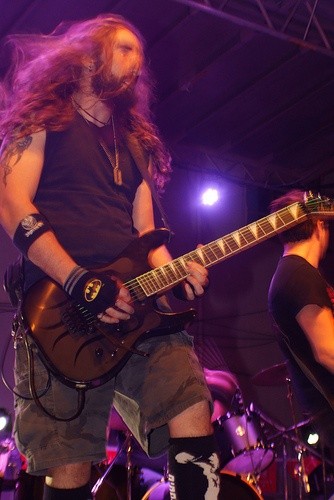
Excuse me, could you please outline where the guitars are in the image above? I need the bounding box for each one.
[20,191,334,391]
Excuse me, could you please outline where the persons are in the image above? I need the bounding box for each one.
[0,15,262,500]
[266,188,334,500]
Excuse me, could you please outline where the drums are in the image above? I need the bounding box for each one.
[212,410,275,475]
[141,469,264,500]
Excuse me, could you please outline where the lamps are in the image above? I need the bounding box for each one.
[0,408,8,430]
[301,425,319,445]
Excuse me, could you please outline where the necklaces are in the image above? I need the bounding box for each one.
[70,91,123,186]
